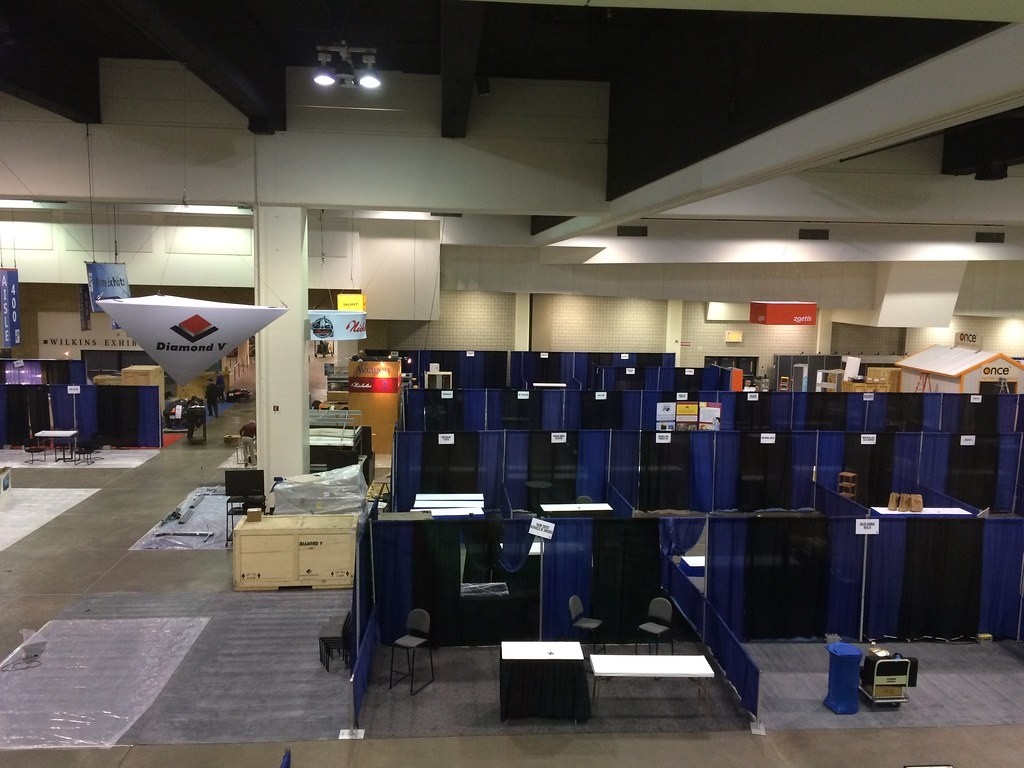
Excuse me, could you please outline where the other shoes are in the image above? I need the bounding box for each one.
[208,414,212,416]
[216,414,218,417]
[245,463,248,467]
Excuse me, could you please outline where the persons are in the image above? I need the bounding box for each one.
[205,371,226,418]
[239,421,257,467]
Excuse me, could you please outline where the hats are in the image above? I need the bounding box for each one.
[208,378,213,382]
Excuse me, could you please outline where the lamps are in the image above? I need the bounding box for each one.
[312,37,384,91]
[473,73,491,97]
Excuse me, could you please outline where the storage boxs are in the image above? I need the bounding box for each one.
[223,434,242,448]
[0,466,11,496]
[862,680,904,699]
[247,508,262,522]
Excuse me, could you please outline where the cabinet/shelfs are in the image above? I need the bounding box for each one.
[225,495,265,548]
[837,470,858,503]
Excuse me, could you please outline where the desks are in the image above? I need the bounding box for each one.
[870,507,972,518]
[35,430,78,463]
[524,480,553,517]
[680,556,706,594]
[588,654,715,722]
[532,382,566,390]
[538,502,613,519]
[499,639,592,726]
[410,493,485,521]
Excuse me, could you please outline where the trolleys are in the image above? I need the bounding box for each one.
[859,658,911,708]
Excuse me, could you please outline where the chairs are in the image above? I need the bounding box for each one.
[319,612,354,672]
[46,428,81,452]
[569,594,607,675]
[576,496,594,504]
[23,439,46,465]
[388,608,436,696]
[74,441,94,465]
[635,596,675,680]
